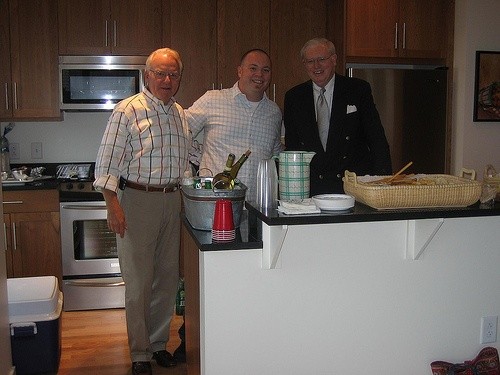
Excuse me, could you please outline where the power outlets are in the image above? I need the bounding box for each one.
[480,315,497,345]
[9,144,20,160]
[31,142,42,159]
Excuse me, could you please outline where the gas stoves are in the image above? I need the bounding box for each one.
[58,178,105,201]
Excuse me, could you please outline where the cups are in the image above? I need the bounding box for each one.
[211,200,236,241]
[256,158,278,208]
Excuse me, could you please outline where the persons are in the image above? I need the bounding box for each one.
[283,37,392,198]
[93,48,194,375]
[183,49,285,210]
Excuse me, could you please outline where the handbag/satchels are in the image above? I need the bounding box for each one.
[430,347,500,375]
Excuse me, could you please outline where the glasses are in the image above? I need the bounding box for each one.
[303,56,331,66]
[149,69,179,81]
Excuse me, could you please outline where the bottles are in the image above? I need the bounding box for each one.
[211,153,235,190]
[231,154,248,180]
[205,179,211,190]
[194,178,202,189]
[176,282,185,315]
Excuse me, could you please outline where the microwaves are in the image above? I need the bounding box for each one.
[58,55,149,112]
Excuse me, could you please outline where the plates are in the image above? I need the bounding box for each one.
[312,194,355,211]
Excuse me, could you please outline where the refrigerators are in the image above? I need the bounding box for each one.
[346,65,449,175]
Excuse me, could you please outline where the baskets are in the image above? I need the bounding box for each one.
[483,165,500,202]
[341,169,482,210]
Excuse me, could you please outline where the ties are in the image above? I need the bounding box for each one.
[316,88,331,152]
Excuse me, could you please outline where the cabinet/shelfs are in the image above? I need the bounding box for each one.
[0,0,456,175]
[2,190,62,291]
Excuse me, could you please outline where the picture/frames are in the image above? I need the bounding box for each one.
[473,50,500,122]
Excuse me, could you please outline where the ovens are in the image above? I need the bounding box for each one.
[60,201,126,312]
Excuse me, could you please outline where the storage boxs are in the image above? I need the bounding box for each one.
[7,276,64,375]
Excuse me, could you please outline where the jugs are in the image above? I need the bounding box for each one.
[272,151,317,201]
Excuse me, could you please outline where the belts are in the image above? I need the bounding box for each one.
[125,180,180,193]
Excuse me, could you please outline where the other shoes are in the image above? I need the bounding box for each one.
[175,341,186,362]
[132,362,152,375]
[152,349,177,366]
